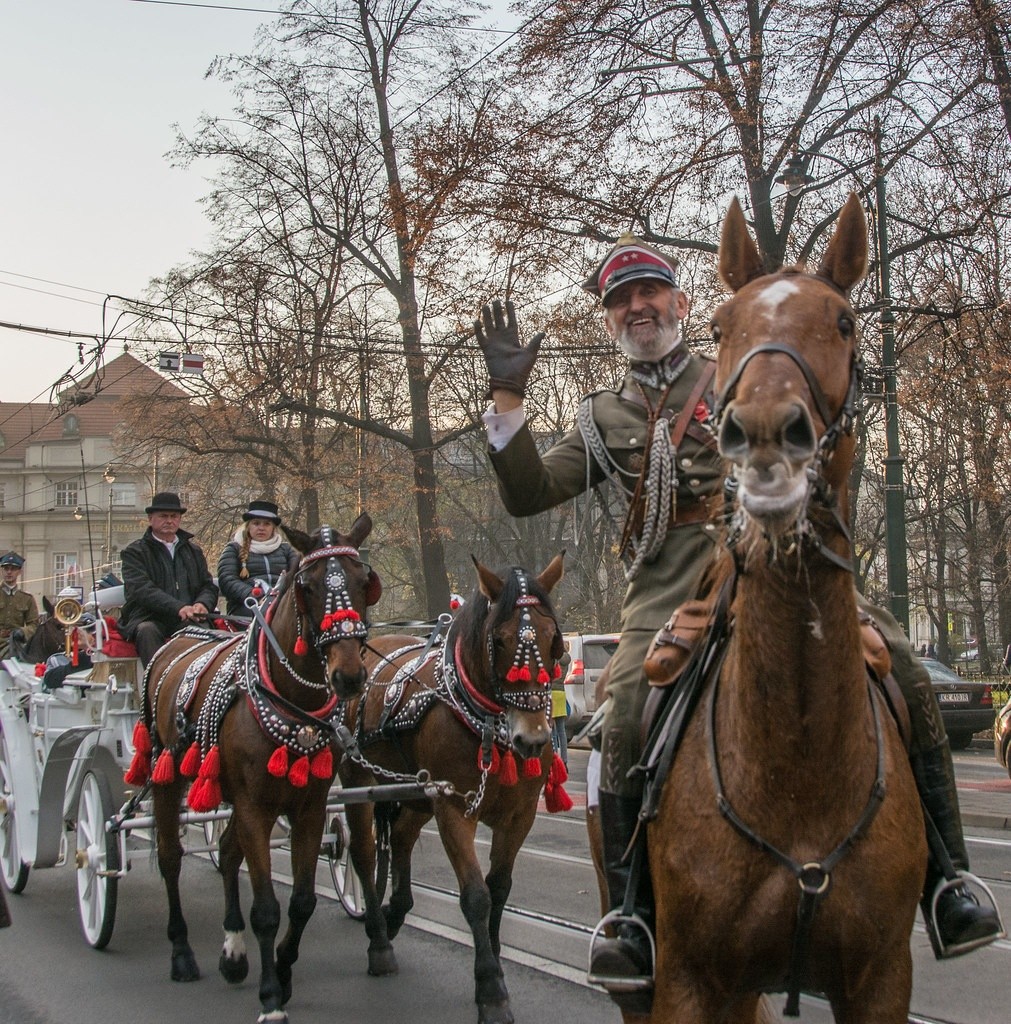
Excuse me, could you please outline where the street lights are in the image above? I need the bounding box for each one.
[773,114,910,642]
[73,487,116,572]
[104,443,160,498]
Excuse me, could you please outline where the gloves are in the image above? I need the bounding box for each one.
[473,299,546,399]
[10,628,25,643]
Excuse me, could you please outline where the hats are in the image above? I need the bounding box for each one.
[0,550,26,568]
[145,491,188,514]
[242,500,283,526]
[94,572,123,588]
[579,231,682,308]
[73,612,97,639]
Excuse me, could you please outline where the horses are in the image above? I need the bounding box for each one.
[584,190,930,1024]
[18,595,66,666]
[138,511,373,1024]
[336,548,567,1024]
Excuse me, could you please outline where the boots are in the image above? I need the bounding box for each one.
[589,786,657,1018]
[907,731,1003,961]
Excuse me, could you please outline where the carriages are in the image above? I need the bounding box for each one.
[1,509,570,1023]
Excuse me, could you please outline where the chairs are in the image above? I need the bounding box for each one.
[89,590,150,708]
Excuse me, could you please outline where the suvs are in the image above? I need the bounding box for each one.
[558,630,623,745]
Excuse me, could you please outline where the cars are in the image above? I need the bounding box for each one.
[960,643,1004,662]
[915,656,998,752]
[948,636,978,653]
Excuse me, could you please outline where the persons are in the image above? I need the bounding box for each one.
[94,573,122,590]
[469,232,1003,1012]
[549,650,570,775]
[115,492,217,668]
[218,500,304,629]
[907,638,954,660]
[0,554,40,662]
